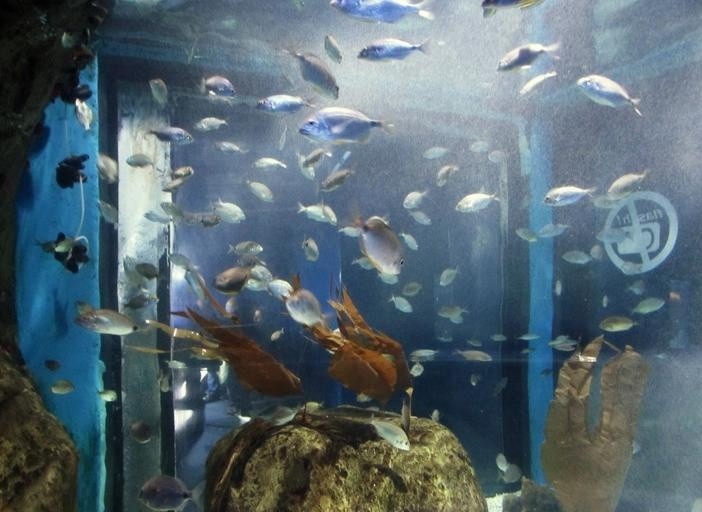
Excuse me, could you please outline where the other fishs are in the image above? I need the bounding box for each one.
[38,71,667,483]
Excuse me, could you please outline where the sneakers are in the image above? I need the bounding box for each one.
[226,401,240,413]
[202,390,221,402]
